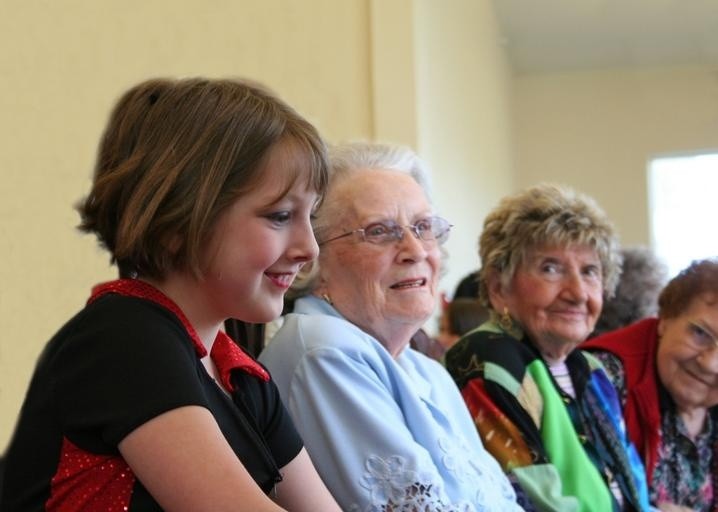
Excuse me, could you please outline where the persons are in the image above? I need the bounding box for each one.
[576,259,718,511]
[441,184,660,512]
[588,243,665,337]
[253,141,526,512]
[1,76,343,512]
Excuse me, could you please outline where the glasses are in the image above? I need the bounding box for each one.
[318,216,454,246]
